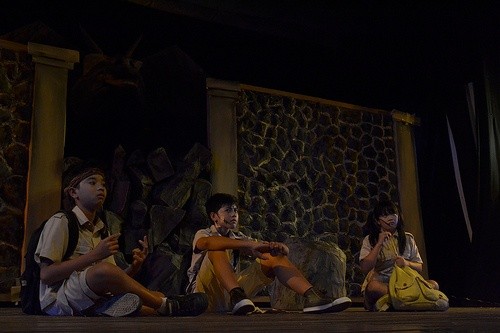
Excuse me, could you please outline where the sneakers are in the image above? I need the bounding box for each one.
[229,288,254,314]
[302,288,351,313]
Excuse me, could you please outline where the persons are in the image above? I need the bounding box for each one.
[34,160,211,320]
[357,199,450,314]
[185,193,352,316]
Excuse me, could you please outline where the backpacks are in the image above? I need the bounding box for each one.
[388,261,439,312]
[19,209,109,314]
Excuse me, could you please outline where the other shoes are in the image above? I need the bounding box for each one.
[375,295,389,310]
[163,292,209,315]
[93,293,142,317]
[437,299,449,310]
